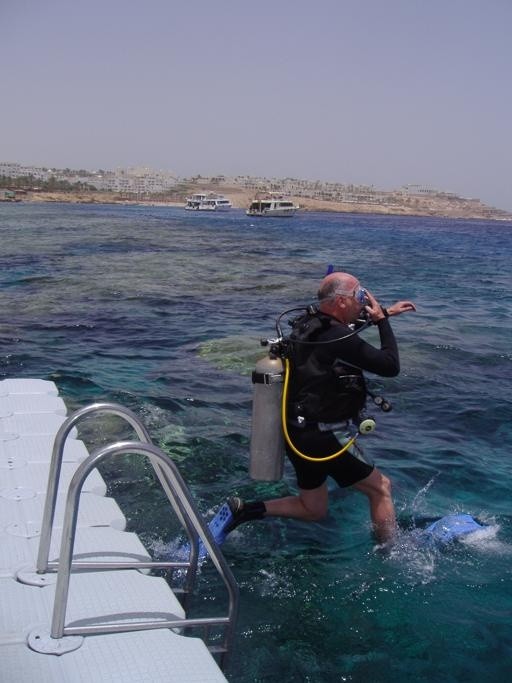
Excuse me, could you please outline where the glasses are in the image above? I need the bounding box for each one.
[332,288,369,306]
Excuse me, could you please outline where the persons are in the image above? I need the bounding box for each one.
[172,269,484,580]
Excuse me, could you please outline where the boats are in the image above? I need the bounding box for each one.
[244,197,299,217]
[185,191,233,213]
[0,188,20,202]
